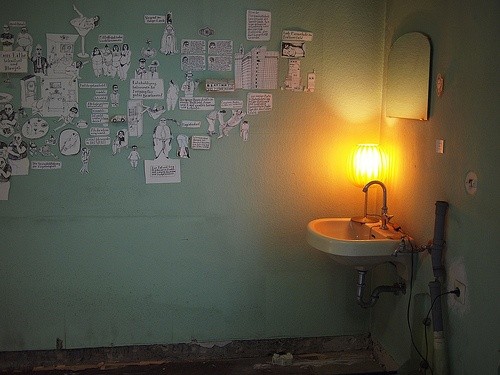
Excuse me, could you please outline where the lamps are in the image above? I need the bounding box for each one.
[352,144,384,224]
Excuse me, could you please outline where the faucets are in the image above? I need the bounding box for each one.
[363,180,388,209]
[392,248,419,256]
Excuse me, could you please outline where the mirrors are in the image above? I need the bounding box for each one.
[386,32,434,121]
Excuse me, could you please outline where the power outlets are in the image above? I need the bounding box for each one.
[452,279,468,306]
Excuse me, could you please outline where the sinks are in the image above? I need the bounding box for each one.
[314,219,386,241]
